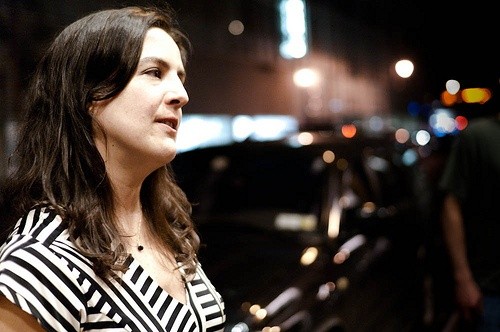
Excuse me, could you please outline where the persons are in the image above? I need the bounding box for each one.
[0,5,227,332]
[441,113,500,332]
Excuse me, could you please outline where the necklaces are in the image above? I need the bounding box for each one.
[123,224,145,251]
